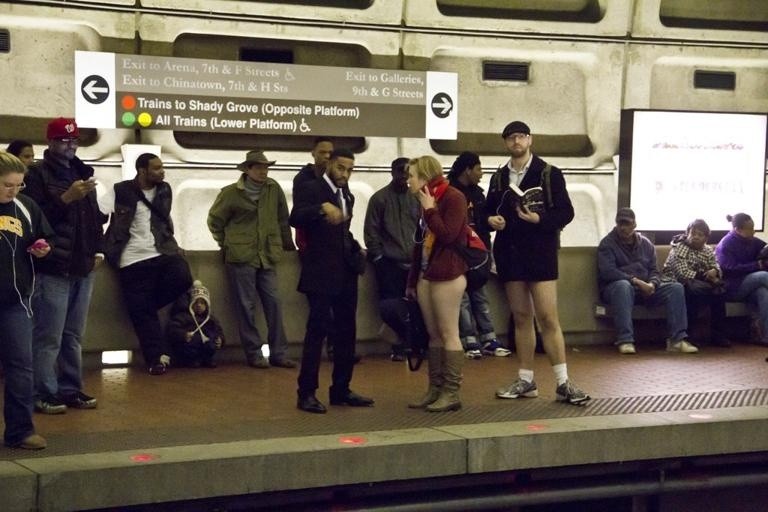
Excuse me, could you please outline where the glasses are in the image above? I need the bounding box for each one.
[505,133,530,142]
[53,137,82,145]
[0,180,27,192]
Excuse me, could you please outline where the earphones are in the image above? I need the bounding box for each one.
[519,170,523,174]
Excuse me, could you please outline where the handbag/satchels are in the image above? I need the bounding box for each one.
[398,290,429,373]
[446,222,493,292]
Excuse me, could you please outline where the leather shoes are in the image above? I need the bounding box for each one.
[329,389,376,406]
[296,389,327,414]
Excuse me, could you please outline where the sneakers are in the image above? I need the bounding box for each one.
[187,359,218,369]
[33,396,68,415]
[15,432,48,450]
[463,343,483,360]
[59,391,98,409]
[495,377,538,400]
[481,338,513,358]
[710,337,730,348]
[555,380,592,406]
[247,355,272,370]
[148,362,169,375]
[617,342,637,356]
[268,358,298,369]
[664,338,699,354]
[389,349,406,362]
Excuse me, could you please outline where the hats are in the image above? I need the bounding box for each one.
[391,158,413,174]
[235,150,278,172]
[614,207,637,224]
[501,120,531,140]
[46,117,81,141]
[187,280,211,312]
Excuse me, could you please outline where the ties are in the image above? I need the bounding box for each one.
[334,188,344,215]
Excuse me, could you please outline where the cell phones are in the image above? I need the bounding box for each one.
[27,239,49,253]
[88,176,96,183]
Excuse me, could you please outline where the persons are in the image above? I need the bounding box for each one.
[207,149,297,370]
[0,117,107,451]
[662,220,731,344]
[446,150,512,358]
[294,139,362,365]
[596,207,699,353]
[184,280,225,368]
[366,158,421,360]
[714,213,768,347]
[102,154,193,374]
[403,156,468,412]
[477,121,591,406]
[291,147,375,412]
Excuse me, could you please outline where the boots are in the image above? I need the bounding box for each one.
[407,347,445,410]
[425,346,465,413]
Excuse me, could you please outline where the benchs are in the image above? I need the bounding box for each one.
[594,302,756,317]
